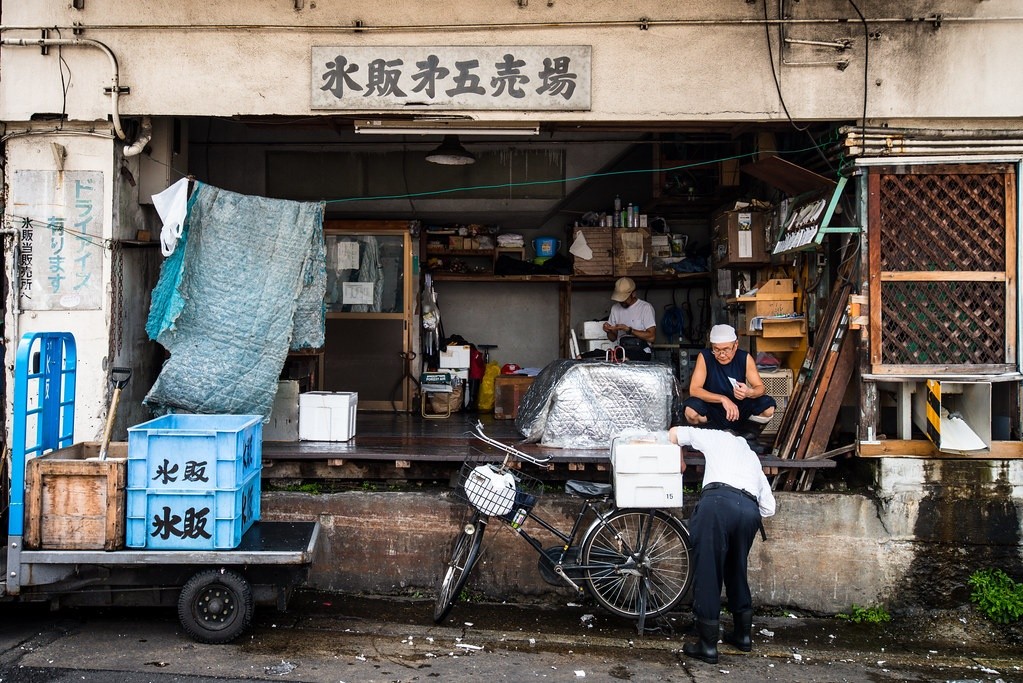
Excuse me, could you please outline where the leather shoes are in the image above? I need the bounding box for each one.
[721,631,751,653]
[682,640,719,663]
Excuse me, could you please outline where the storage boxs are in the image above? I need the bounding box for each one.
[298,389,358,442]
[126,414,268,551]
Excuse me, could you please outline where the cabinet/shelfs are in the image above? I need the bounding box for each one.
[277,215,426,415]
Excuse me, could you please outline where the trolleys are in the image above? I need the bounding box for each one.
[2,329,326,643]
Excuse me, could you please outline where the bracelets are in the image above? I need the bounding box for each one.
[626,327,632,335]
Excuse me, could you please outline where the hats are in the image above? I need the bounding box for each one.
[610,277,635,301]
[711,324,737,343]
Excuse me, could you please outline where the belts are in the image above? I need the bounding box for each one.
[698,482,760,502]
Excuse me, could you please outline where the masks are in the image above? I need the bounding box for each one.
[619,301,630,308]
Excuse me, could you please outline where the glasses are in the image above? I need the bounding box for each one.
[711,342,736,354]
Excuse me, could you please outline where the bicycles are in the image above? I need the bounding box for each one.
[427,417,693,638]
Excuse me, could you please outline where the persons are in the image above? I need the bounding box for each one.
[681,324,776,453]
[667,427,776,665]
[576,278,657,361]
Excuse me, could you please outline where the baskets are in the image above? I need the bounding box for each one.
[452,445,542,528]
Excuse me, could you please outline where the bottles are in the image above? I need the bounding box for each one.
[599,195,641,228]
